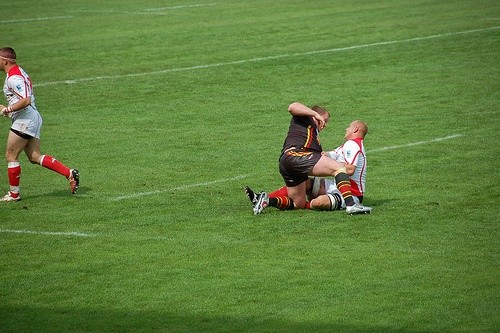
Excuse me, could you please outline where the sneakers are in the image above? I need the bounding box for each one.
[253,192,269,215]
[0,191,21,202]
[244,186,263,206]
[68,168,80,194]
[346,204,373,215]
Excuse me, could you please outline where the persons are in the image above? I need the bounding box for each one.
[253,102,373,215]
[0,47,79,202]
[245,120,368,210]
